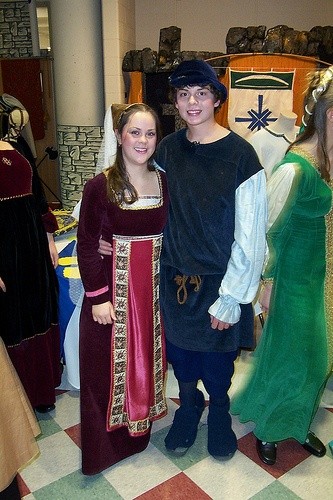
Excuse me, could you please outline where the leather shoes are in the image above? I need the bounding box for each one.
[302,432,326,457]
[256,438,277,465]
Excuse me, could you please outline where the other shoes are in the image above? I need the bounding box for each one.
[208,396,237,461]
[164,389,204,452]
[36,403,55,413]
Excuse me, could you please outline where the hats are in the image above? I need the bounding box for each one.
[111,103,145,130]
[168,60,227,104]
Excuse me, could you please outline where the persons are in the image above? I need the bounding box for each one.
[228,69,333,466]
[0,139,60,414]
[154,58,268,462]
[76,103,171,476]
